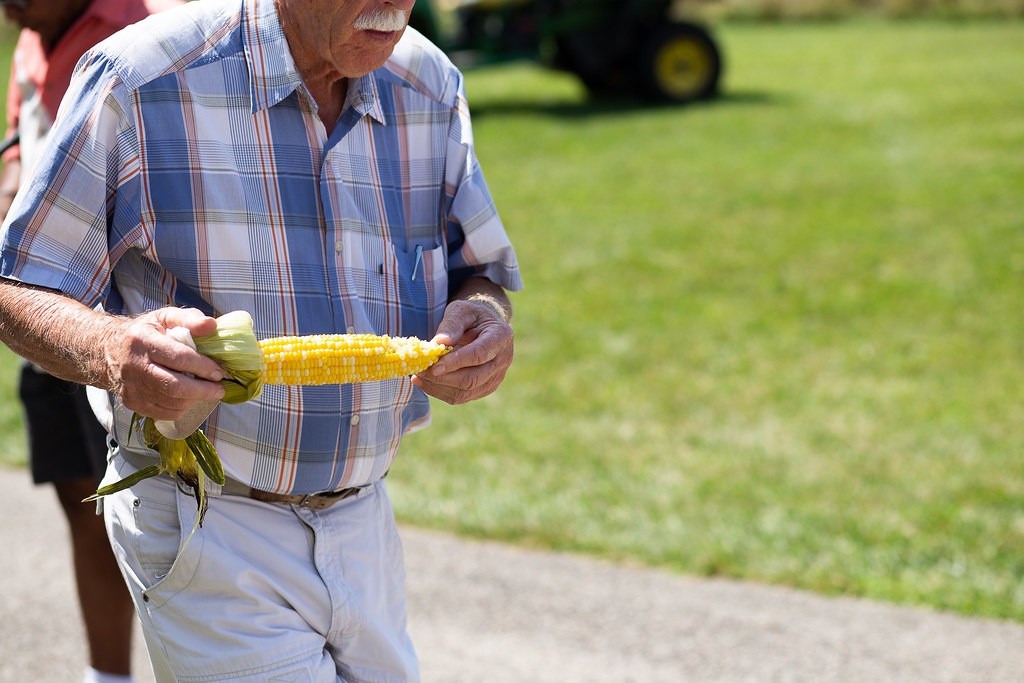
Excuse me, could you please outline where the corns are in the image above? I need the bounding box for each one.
[255,334,453,386]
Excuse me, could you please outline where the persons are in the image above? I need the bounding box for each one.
[1,1,188,682]
[1,0,522,682]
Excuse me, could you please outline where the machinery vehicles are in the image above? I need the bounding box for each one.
[412,1,724,110]
